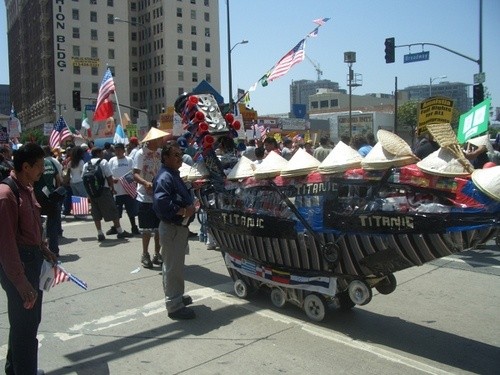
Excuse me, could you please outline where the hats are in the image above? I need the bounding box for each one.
[174,141,500,199]
[141,126,171,142]
[129,137,138,144]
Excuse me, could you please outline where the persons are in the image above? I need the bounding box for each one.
[152,141,194,319]
[0,133,142,257]
[131,126,170,267]
[177,132,246,249]
[243,133,377,162]
[0,142,56,375]
[414,126,500,168]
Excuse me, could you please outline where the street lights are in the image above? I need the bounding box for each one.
[430,76,447,97]
[114,18,151,132]
[52,101,67,118]
[228,41,248,113]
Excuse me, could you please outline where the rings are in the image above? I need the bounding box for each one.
[30,292,32,294]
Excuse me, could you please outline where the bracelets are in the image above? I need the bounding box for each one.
[182,208,186,217]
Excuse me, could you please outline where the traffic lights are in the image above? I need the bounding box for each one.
[73,90,81,108]
[473,85,483,106]
[384,38,395,63]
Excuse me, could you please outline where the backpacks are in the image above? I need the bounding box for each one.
[83,159,106,200]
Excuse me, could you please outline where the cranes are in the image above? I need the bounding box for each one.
[305,54,323,80]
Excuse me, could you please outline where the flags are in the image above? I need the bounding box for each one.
[120,171,138,199]
[52,266,87,289]
[92,67,115,121]
[71,196,89,215]
[267,39,305,80]
[82,109,91,137]
[307,18,330,38]
[50,117,72,149]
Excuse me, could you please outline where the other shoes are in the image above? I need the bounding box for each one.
[168,307,195,320]
[105,226,121,235]
[140,254,153,268]
[131,226,140,235]
[205,244,216,250]
[152,253,163,265]
[97,233,105,242]
[183,295,192,306]
[187,232,198,238]
[118,231,134,239]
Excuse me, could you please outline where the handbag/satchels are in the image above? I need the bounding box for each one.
[47,185,67,211]
